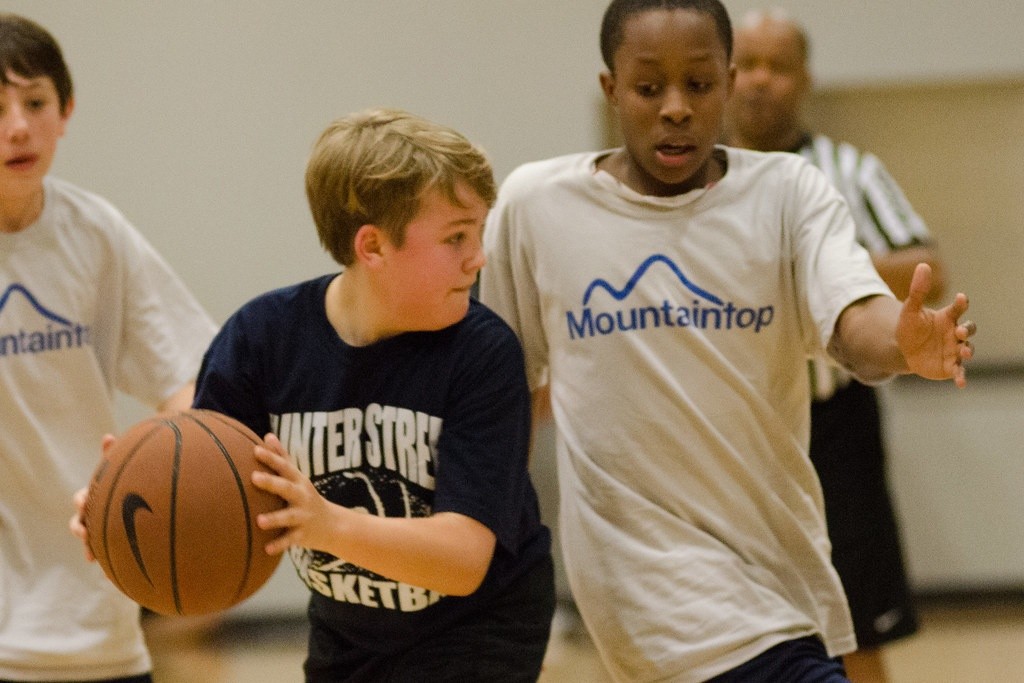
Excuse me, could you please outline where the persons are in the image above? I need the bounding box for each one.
[479,0,978,683]
[721,16,949,682]
[70,111,558,682]
[0,14,220,683]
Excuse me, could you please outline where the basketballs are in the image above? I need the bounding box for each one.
[80,409,287,618]
